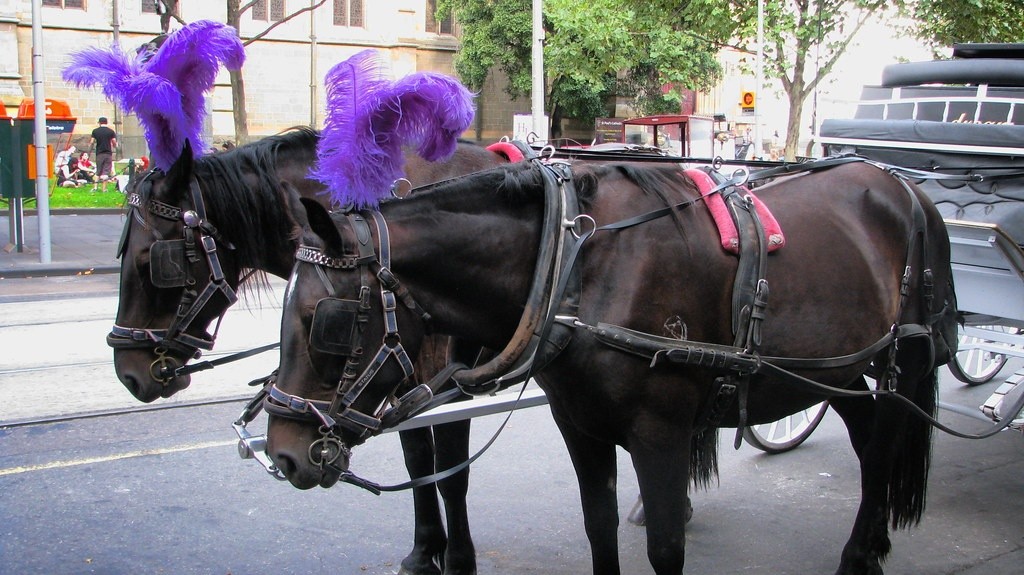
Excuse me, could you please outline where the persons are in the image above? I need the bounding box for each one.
[644,129,671,150]
[205,142,234,154]
[55,118,117,192]
[766,148,785,161]
[137,156,148,175]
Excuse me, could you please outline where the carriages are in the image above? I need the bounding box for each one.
[58,17,1024,575]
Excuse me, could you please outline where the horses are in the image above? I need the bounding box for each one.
[113,135,695,575]
[263,158,958,575]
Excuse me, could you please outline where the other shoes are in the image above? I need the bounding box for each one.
[90,188,97,192]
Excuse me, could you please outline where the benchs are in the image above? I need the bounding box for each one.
[819,57,1024,177]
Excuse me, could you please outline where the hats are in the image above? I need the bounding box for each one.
[97,117,107,122]
